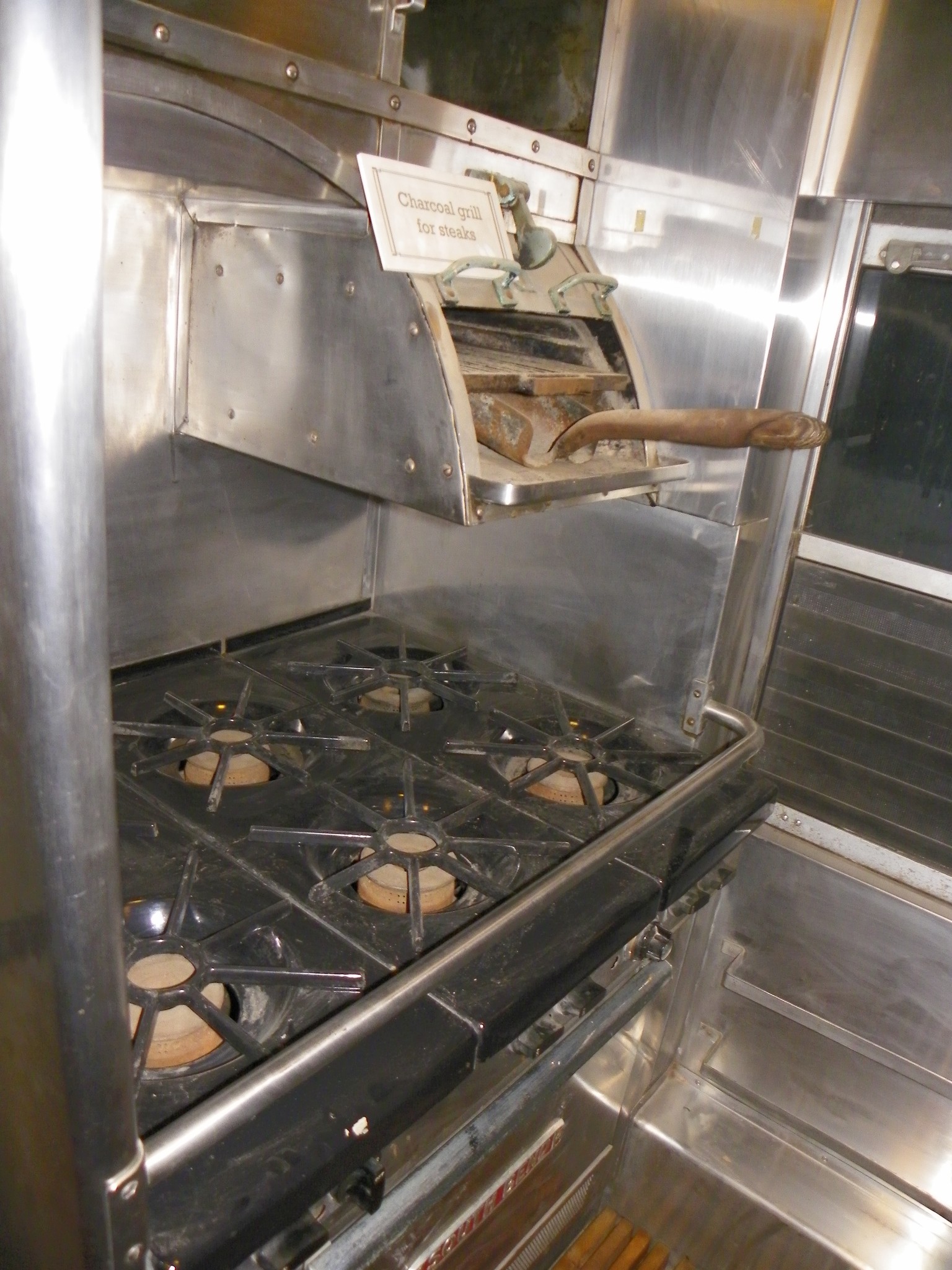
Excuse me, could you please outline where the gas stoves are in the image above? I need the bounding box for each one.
[87,592,779,1270]
